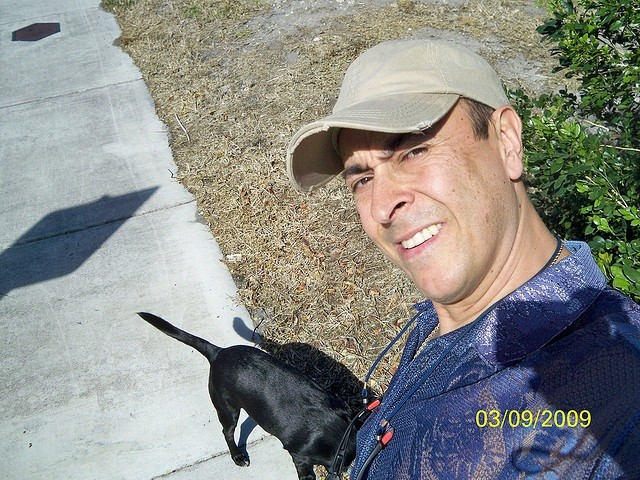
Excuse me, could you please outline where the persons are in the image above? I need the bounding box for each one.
[286,38,640,480]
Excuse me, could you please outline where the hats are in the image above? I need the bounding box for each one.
[285,35,513,197]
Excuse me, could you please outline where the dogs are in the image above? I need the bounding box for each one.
[131,310,357,480]
